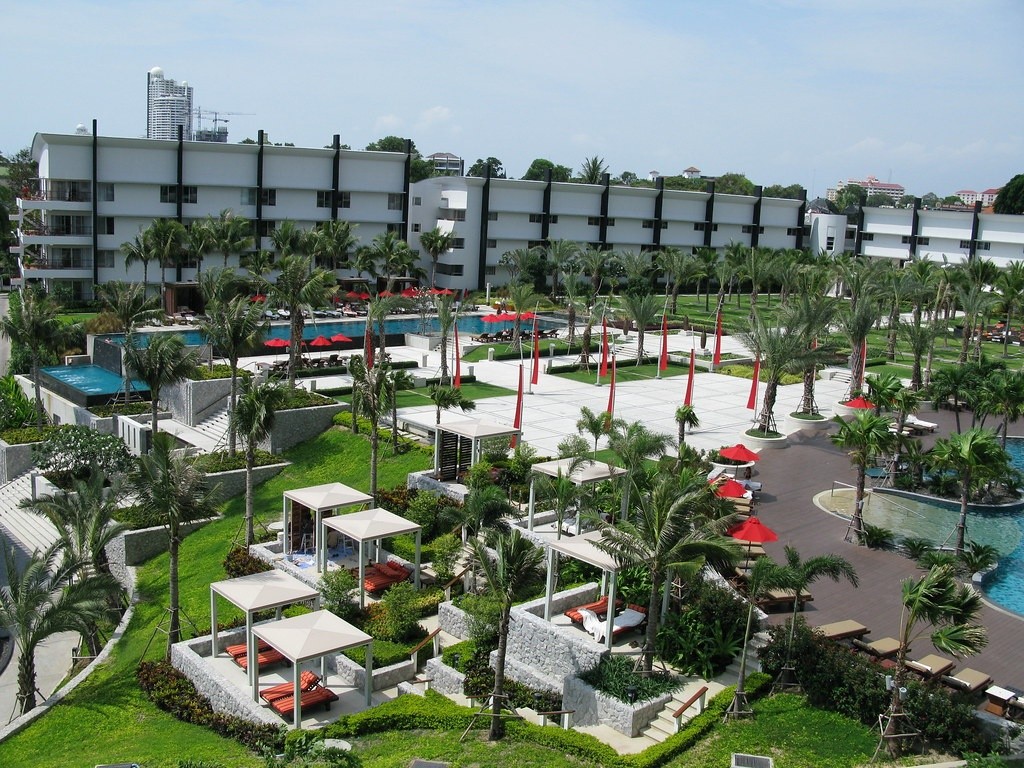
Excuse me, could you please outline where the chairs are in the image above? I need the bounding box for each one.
[469,328,561,344]
[253,351,391,372]
[258,670,322,706]
[597,603,647,643]
[350,560,414,595]
[270,684,340,723]
[225,637,273,661]
[145,298,479,326]
[714,475,1024,724]
[885,413,941,436]
[564,596,623,627]
[234,648,291,677]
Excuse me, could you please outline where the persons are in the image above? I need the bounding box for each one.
[497,296,508,314]
[743,467,753,490]
[327,528,337,548]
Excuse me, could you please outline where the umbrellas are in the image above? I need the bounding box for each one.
[250,294,266,303]
[335,285,455,299]
[708,479,747,497]
[263,333,353,366]
[480,312,539,335]
[725,516,779,573]
[719,444,760,482]
[845,396,877,424]
